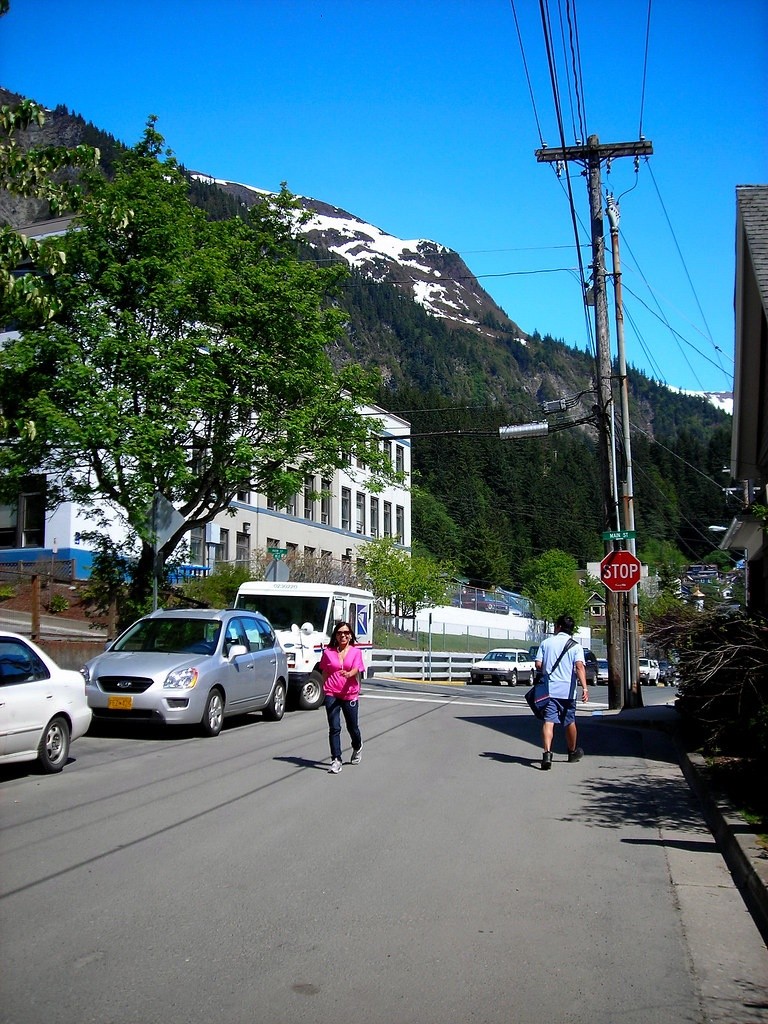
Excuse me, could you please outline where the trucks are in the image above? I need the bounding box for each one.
[230,581,377,711]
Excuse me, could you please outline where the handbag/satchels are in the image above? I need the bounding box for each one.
[525,687,544,721]
[535,674,549,709]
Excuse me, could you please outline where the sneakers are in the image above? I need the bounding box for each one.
[568,747,584,762]
[351,743,363,764]
[327,758,342,774]
[542,752,553,770]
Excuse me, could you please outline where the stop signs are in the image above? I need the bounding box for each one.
[598,550,642,592]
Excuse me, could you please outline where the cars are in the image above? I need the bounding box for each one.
[595,658,609,684]
[448,591,535,619]
[0,633,92,775]
[471,646,537,687]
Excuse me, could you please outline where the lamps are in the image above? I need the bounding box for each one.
[346,547,352,556]
[243,522,250,530]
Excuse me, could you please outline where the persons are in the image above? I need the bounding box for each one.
[535,616,589,770]
[319,622,364,772]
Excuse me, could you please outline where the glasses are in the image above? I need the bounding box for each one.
[336,631,350,635]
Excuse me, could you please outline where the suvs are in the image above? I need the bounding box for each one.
[78,608,288,739]
[658,660,676,688]
[637,658,660,687]
[577,647,599,687]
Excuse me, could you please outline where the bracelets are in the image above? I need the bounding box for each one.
[583,689,588,692]
